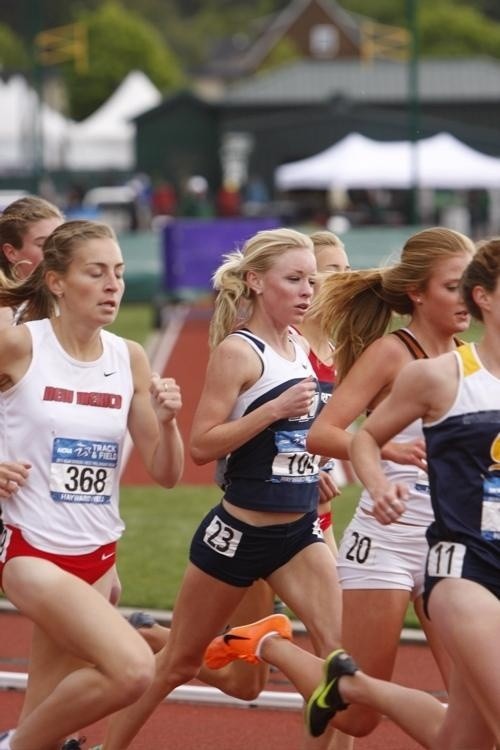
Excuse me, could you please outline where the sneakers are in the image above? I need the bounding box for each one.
[129,612,156,629]
[203,614,294,669]
[305,647,359,738]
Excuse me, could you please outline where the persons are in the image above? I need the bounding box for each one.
[304,237,500,750]
[35,169,423,232]
[204,228,476,737]
[0,221,184,750]
[0,198,121,750]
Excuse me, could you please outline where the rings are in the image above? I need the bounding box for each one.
[385,508,393,514]
[391,499,400,508]
[164,383,168,392]
